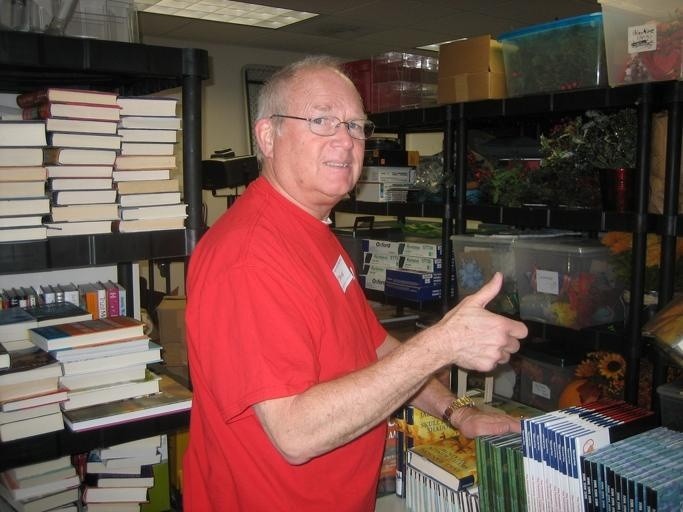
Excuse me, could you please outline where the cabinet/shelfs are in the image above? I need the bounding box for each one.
[328,80,683,412]
[0,26,209,511]
[322,80,682,499]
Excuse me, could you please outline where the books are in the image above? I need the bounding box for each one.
[0,86,190,243]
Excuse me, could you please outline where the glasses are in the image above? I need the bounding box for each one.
[270,112,378,141]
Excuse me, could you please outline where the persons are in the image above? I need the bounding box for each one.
[165,53,529,512]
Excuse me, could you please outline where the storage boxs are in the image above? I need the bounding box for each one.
[493,339,576,412]
[436,0,683,104]
[657,383,682,430]
[359,236,454,301]
[498,158,544,173]
[155,293,188,369]
[449,230,633,329]
[336,48,439,113]
[355,138,418,203]
